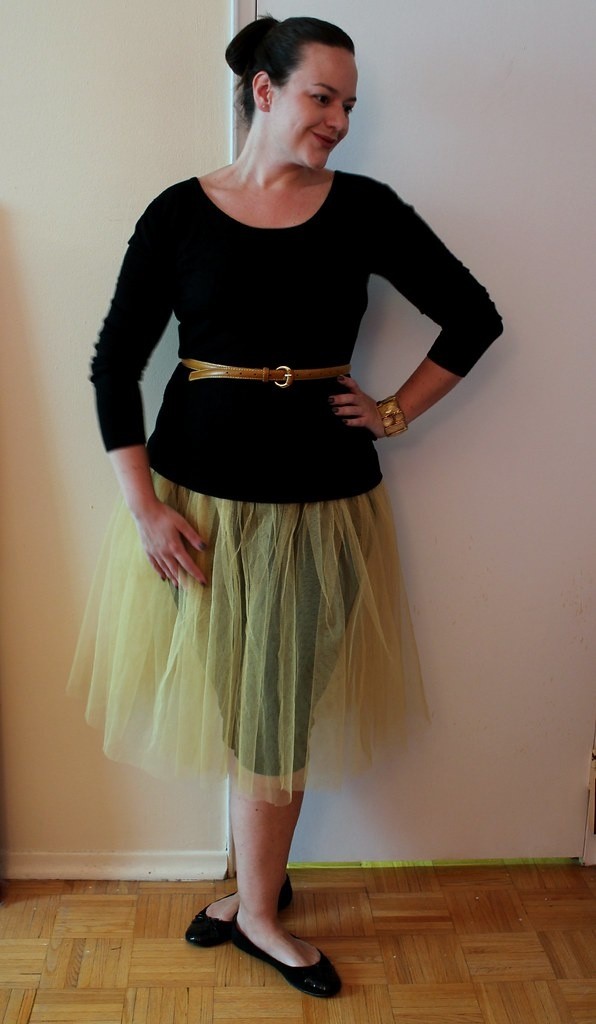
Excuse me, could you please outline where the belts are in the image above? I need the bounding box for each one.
[182,359,351,387]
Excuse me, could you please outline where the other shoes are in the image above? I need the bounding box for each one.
[229,912,342,997]
[186,873,294,947]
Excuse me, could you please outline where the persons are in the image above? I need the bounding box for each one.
[67,13,506,998]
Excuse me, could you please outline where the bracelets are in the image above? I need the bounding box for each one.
[375,396,408,438]
